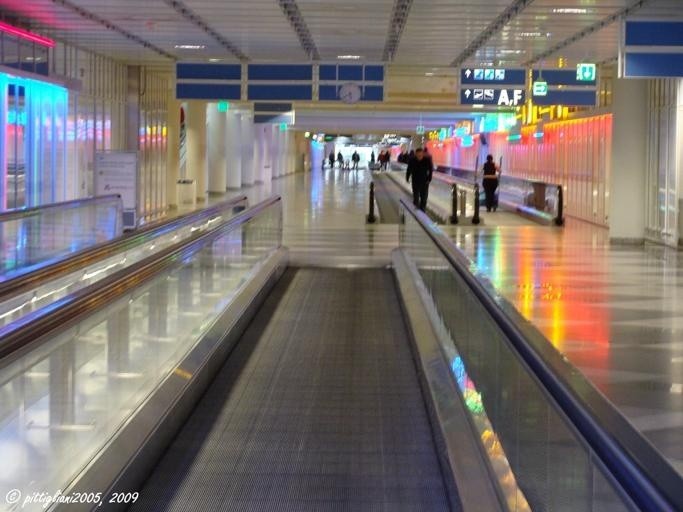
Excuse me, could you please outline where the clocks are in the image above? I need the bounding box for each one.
[339,83,361,104]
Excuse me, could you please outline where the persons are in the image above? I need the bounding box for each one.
[329,147,432,212]
